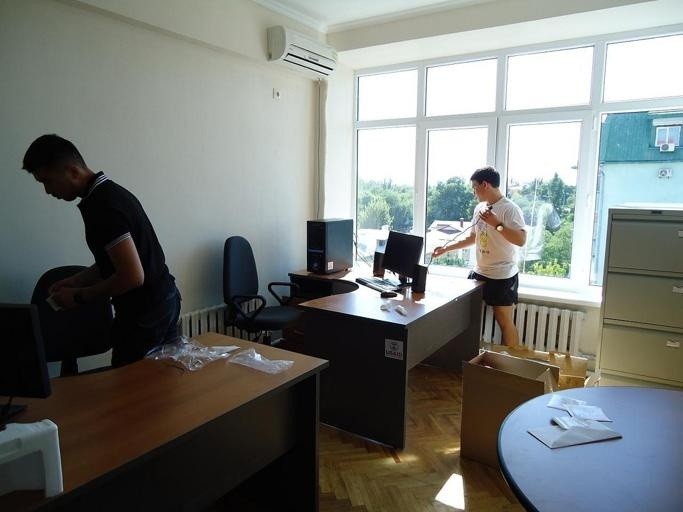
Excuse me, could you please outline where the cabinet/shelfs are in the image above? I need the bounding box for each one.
[593,206,682,388]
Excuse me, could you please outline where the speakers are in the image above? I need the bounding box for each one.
[412,264,428,293]
[373,251,385,277]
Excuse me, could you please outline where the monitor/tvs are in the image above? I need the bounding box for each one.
[381,230,423,287]
[0,302,53,421]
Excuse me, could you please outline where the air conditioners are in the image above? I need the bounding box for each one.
[266,24,338,81]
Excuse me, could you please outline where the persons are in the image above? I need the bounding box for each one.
[20,133,183,363]
[431,166,528,346]
[520,202,561,272]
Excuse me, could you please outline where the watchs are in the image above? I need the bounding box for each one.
[495,222,505,232]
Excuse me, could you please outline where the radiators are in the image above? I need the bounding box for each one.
[178,293,262,345]
[480,302,585,361]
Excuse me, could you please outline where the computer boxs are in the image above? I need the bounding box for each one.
[307,218,353,274]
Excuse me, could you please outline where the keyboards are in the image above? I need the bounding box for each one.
[356,277,400,291]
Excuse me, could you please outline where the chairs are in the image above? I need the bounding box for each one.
[28,263,114,377]
[220,233,307,354]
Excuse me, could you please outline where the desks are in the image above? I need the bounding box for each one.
[1,331,332,512]
[283,264,485,452]
[495,385,682,509]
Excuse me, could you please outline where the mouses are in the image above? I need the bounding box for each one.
[381,292,397,298]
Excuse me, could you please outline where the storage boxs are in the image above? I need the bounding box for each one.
[457,348,560,470]
[480,340,597,389]
